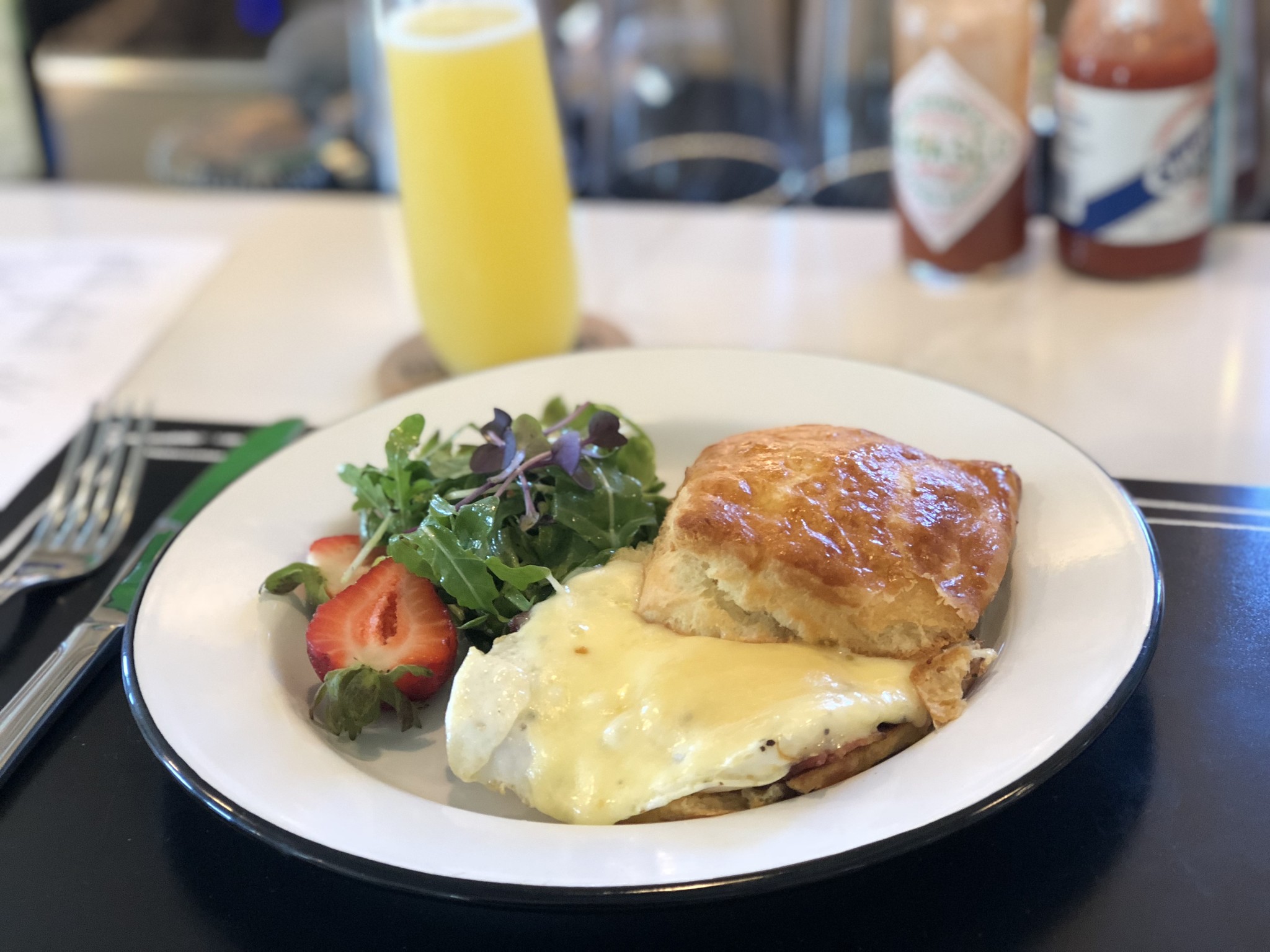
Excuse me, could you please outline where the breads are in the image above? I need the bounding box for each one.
[635,424,1024,730]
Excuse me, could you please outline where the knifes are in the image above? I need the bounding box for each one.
[1,418,310,777]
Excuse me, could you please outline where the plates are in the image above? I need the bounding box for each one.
[118,336,1162,915]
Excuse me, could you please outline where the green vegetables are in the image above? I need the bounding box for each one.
[256,393,672,742]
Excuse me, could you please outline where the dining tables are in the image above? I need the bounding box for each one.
[2,178,1270,952]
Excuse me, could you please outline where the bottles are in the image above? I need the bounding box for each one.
[1051,0,1218,277]
[891,1,1035,278]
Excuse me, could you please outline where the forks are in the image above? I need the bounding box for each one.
[1,397,150,605]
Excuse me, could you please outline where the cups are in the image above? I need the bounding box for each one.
[364,0,584,380]
